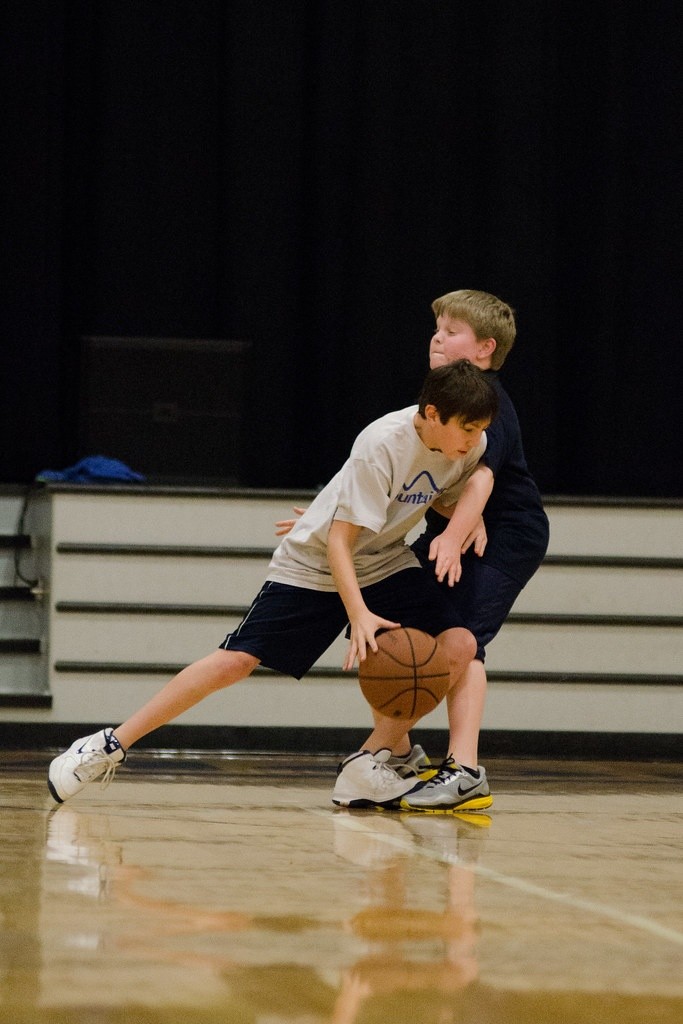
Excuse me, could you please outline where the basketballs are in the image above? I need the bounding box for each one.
[357,627,451,721]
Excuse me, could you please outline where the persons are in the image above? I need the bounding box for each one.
[274,292,549,814]
[45,358,498,805]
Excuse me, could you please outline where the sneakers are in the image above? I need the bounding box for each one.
[400,753,493,811]
[47,728,128,803]
[332,748,426,809]
[385,744,438,782]
